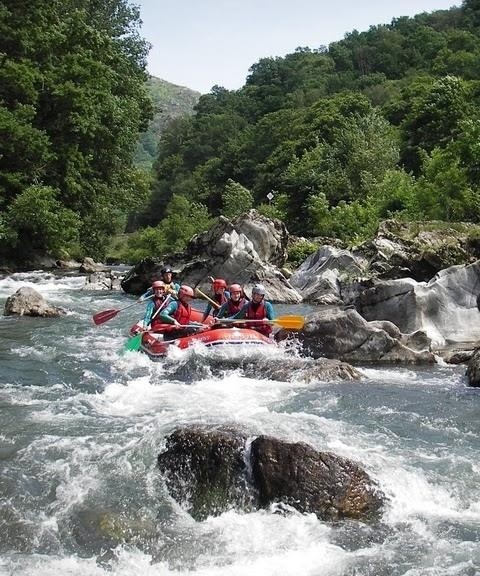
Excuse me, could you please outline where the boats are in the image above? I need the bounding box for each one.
[131,314,278,361]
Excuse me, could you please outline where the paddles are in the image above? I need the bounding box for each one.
[93,294,156,325]
[125,294,172,351]
[217,315,304,328]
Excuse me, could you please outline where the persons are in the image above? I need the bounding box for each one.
[207,283,249,328]
[136,267,181,302]
[208,284,275,338]
[200,278,231,324]
[141,279,179,335]
[159,285,209,341]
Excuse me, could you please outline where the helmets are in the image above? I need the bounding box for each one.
[229,284,242,297]
[152,281,165,293]
[161,268,172,273]
[214,279,226,291]
[179,285,193,298]
[252,284,266,297]
